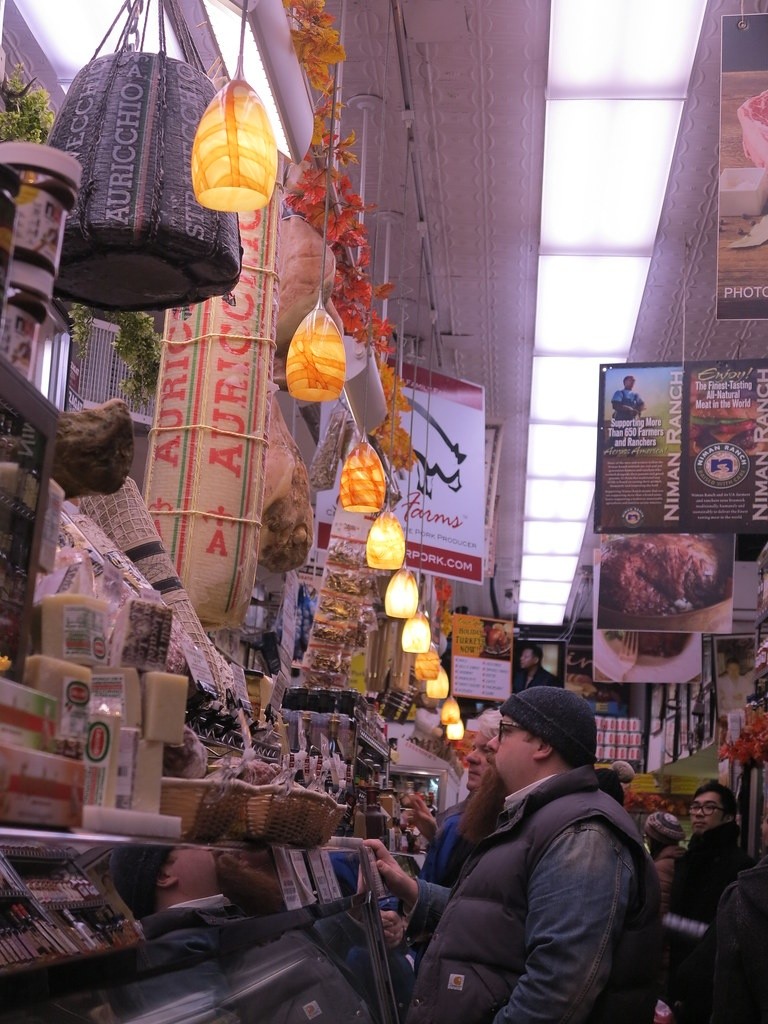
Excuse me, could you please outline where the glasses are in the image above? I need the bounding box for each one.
[690,804,730,817]
[499,719,523,741]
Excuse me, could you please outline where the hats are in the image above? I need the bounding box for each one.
[500,685,598,770]
[644,812,686,846]
[594,761,634,808]
[110,844,176,918]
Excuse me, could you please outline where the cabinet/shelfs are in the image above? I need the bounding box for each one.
[0,355,60,682]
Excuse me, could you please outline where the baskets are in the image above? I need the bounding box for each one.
[160,776,254,840]
[246,782,350,848]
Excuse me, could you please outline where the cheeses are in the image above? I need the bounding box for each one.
[0,461,191,838]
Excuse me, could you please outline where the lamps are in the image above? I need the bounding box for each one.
[366,124,430,570]
[286,1,346,400]
[441,581,462,726]
[341,0,385,513]
[447,721,464,741]
[190,0,278,212]
[385,223,421,621]
[343,90,388,436]
[401,575,433,656]
[427,664,449,698]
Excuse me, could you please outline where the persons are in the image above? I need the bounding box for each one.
[609,376,646,457]
[654,784,757,1024]
[718,658,755,709]
[514,646,556,693]
[113,846,373,1024]
[708,855,768,1024]
[327,851,415,1024]
[363,686,661,1024]
[644,811,687,929]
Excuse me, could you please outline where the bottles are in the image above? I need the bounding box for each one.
[405,781,415,796]
[366,786,384,845]
[328,714,343,761]
[301,712,313,752]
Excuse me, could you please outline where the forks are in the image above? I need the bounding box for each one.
[617,631,639,674]
